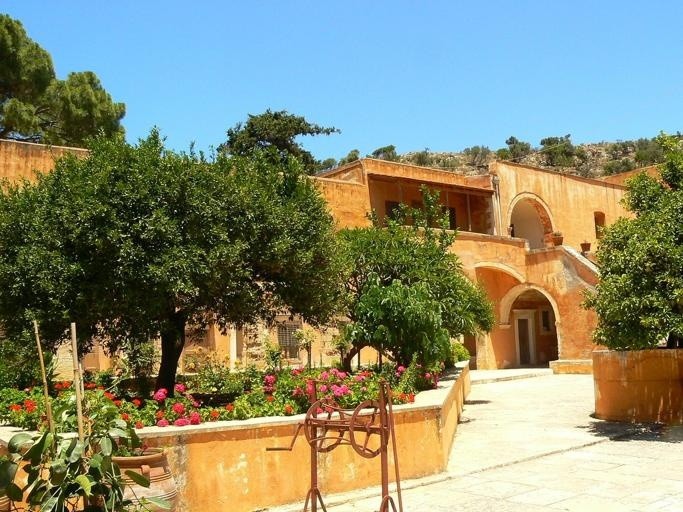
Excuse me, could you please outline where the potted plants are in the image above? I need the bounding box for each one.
[551,231,564,246]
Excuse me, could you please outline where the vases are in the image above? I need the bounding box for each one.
[580,242,592,252]
[95,446,180,512]
[0,444,13,511]
